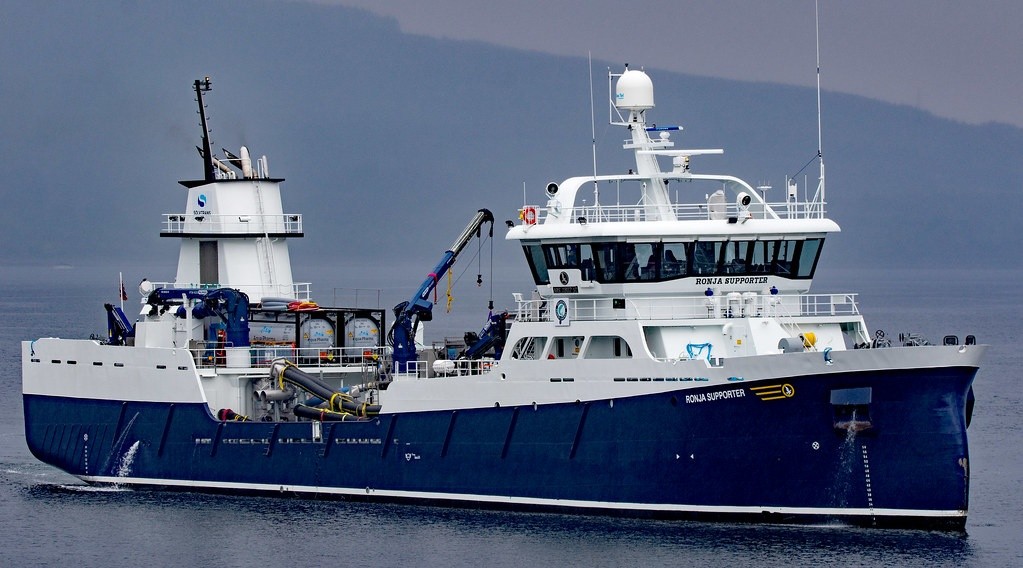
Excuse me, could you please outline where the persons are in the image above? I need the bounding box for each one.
[568,250,577,266]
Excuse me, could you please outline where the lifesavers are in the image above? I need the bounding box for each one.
[289,302,318,310]
[523,207,535,225]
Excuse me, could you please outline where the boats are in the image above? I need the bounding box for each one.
[19,0,992,537]
